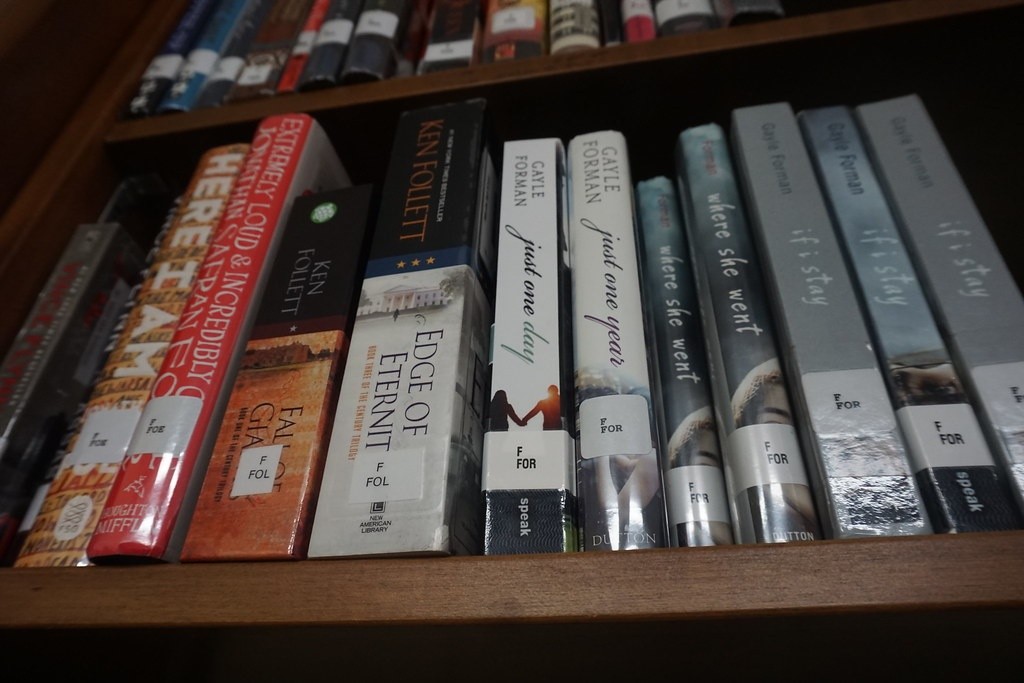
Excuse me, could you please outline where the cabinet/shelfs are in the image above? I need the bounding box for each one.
[0,0,1024,683]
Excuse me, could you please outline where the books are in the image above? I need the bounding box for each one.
[0,0,1024,568]
[304,96,503,559]
